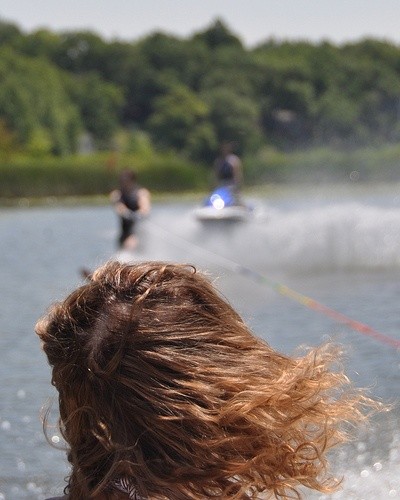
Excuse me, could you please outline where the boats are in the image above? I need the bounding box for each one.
[191,196,266,222]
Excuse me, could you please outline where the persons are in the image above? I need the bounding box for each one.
[108,166,151,252]
[32,258,393,500]
[213,138,244,206]
